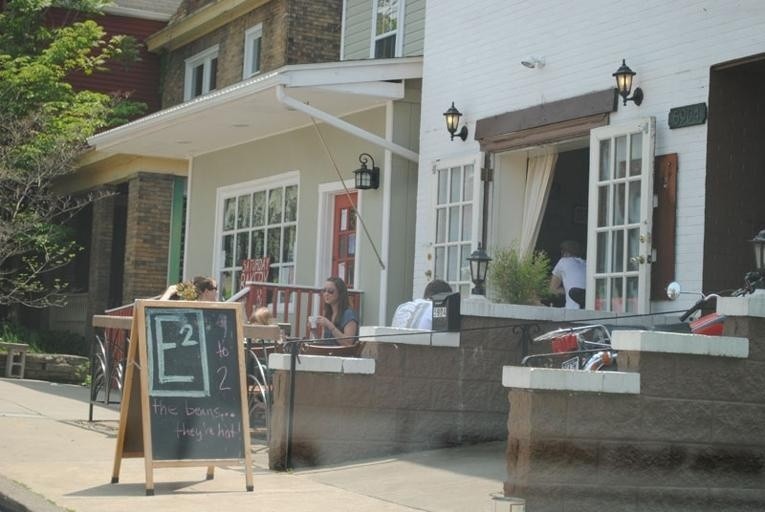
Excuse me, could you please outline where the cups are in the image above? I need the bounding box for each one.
[277,322,291,336]
[307,315,320,331]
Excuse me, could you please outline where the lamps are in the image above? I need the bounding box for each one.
[353,152,380,191]
[441,102,469,143]
[520,55,546,70]
[613,60,646,106]
[465,241,493,296]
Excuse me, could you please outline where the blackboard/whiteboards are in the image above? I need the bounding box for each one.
[137,299,252,467]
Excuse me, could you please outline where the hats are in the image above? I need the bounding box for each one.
[559,240,583,255]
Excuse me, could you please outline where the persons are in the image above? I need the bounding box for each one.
[290,277,359,354]
[550,239,586,308]
[391,278,453,330]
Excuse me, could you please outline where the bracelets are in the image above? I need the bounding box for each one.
[327,322,335,331]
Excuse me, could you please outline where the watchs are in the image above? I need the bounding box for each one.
[190,274,217,300]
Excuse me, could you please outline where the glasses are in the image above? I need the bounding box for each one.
[322,287,335,294]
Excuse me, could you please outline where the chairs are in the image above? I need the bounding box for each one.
[238,322,367,439]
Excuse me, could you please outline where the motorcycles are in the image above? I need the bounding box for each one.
[517,265,764,375]
